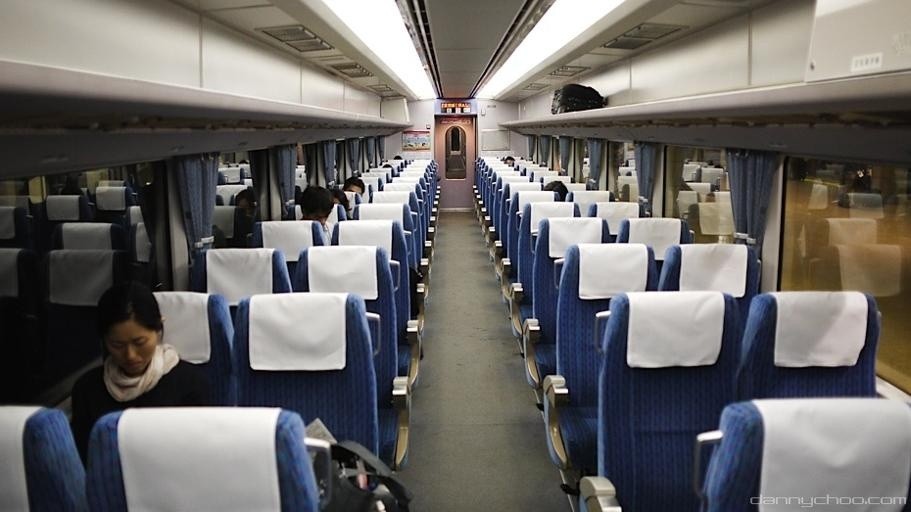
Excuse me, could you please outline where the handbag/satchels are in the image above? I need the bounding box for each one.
[313,439,414,512]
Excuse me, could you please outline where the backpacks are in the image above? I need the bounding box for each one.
[551,83,604,115]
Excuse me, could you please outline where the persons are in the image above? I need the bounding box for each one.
[544,181,568,201]
[330,189,349,211]
[236,190,255,210]
[382,164,392,168]
[342,177,365,198]
[506,156,516,165]
[299,185,333,226]
[70,281,210,440]
[394,156,403,160]
